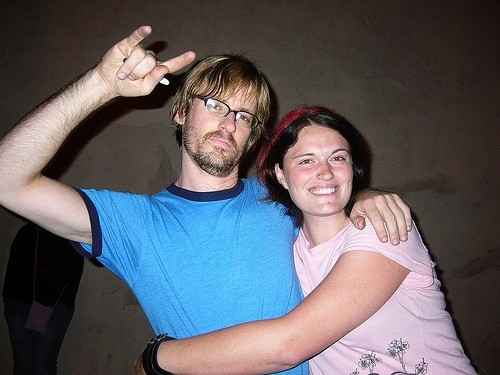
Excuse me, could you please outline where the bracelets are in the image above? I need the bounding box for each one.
[141,333,177,375]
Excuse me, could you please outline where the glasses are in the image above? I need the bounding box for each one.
[190,92,262,128]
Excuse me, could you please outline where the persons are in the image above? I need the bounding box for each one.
[0,25,413,375]
[133,105,480,375]
[1,223,85,375]
